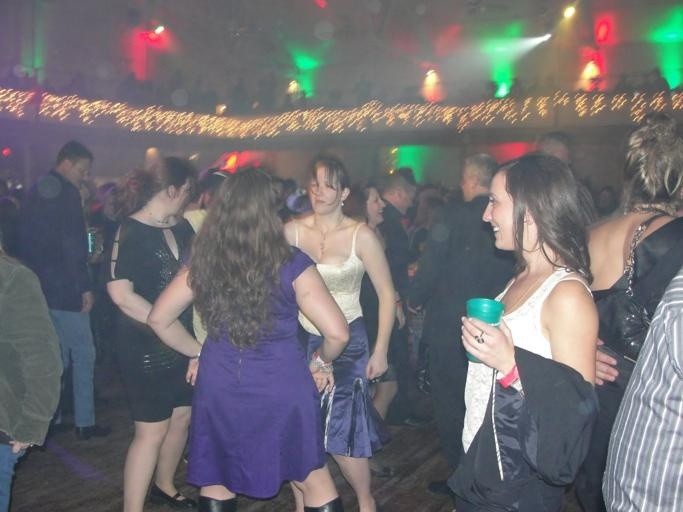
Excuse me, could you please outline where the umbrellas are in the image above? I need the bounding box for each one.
[76,426,111,440]
[48,423,73,437]
[150,481,196,509]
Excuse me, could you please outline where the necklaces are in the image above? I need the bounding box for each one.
[503,270,550,315]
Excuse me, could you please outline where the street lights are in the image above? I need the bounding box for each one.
[596,291,651,388]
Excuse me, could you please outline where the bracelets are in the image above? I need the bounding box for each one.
[190,352,201,359]
[496,364,518,388]
[312,351,332,368]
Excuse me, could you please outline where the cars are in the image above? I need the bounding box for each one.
[463,297,506,362]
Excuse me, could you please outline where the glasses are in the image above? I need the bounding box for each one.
[148,21,165,36]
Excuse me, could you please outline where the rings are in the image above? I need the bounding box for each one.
[475,332,485,344]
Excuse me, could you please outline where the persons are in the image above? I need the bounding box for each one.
[275,134,597,492]
[601,269,682,511]
[279,154,396,511]
[2,140,231,511]
[144,168,352,512]
[446,153,599,511]
[588,108,683,470]
